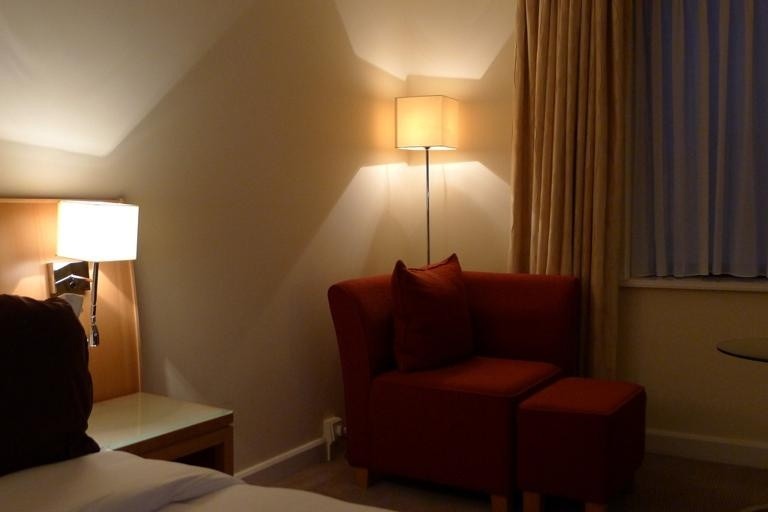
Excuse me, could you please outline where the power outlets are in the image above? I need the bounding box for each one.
[323,415,344,443]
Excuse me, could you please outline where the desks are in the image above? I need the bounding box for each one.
[716,336,768,511]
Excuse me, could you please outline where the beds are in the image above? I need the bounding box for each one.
[0,193,394,511]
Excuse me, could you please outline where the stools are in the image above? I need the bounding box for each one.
[516,376,646,512]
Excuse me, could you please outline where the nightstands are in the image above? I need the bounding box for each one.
[87,390,235,479]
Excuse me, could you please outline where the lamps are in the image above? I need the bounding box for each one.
[395,94,460,266]
[45,198,141,350]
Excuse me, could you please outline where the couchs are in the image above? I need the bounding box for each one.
[327,264,584,511]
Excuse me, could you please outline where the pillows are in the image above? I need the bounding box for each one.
[394,256,481,373]
[0,297,94,473]
